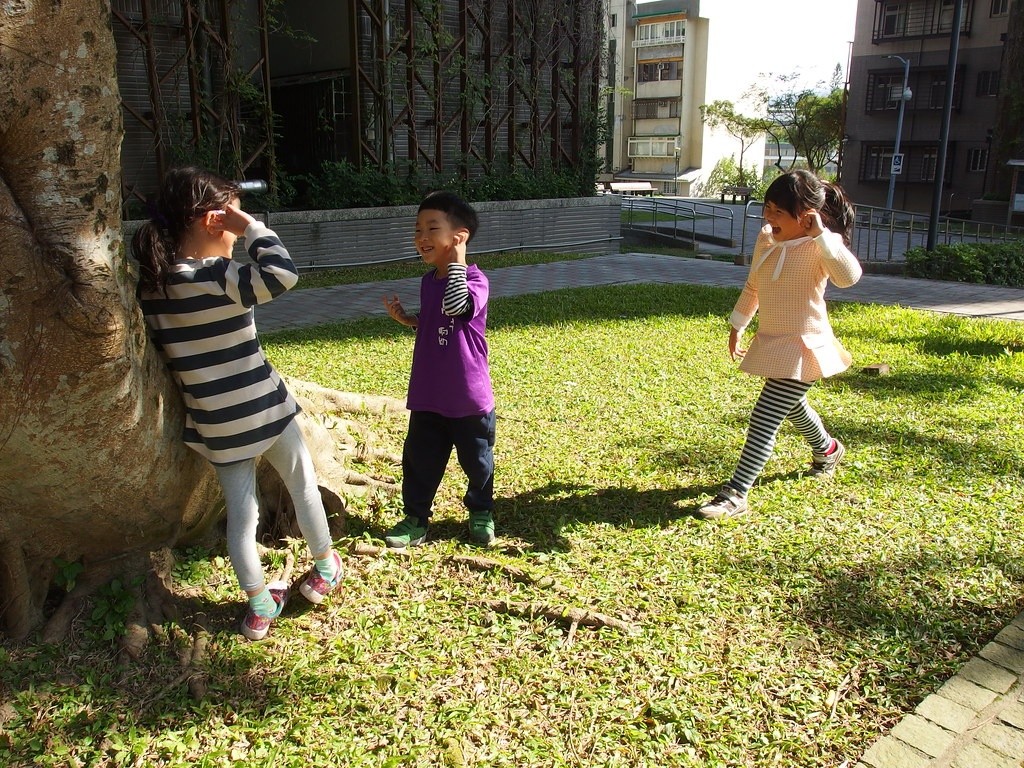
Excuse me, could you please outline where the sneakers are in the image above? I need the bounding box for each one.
[385,515,428,547]
[240,580,292,641]
[694,484,749,520]
[299,552,343,604]
[806,438,845,480]
[468,510,496,545]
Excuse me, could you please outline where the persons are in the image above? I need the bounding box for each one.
[383,191,496,550]
[696,170,862,520]
[132,167,344,640]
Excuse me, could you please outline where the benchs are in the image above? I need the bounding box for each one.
[717,186,755,205]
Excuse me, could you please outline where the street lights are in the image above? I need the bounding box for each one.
[674,146,682,196]
[880,54,909,224]
